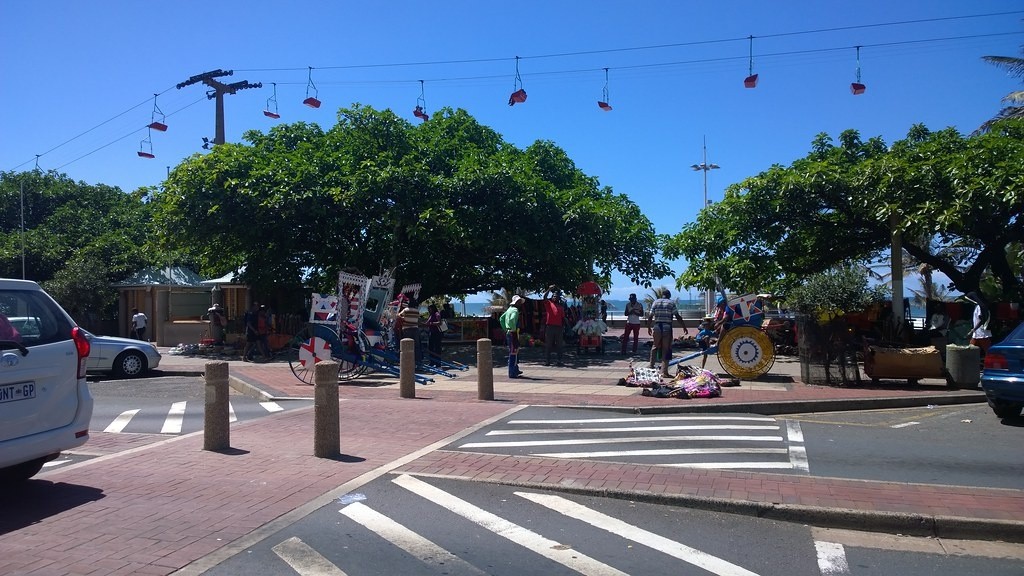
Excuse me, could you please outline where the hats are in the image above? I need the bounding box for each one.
[703,314,715,319]
[716,296,726,307]
[208,304,224,311]
[509,295,525,305]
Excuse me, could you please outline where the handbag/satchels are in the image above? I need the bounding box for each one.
[438,320,449,333]
[220,316,227,326]
[130,329,137,339]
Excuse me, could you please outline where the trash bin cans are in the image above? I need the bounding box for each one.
[946,345,980,389]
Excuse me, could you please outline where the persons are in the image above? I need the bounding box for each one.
[420,305,444,370]
[620,294,644,355]
[242,304,276,362]
[543,285,565,366]
[696,297,734,372]
[964,292,993,354]
[499,295,525,379]
[599,300,607,332]
[397,298,424,371]
[130,308,147,341]
[647,290,688,378]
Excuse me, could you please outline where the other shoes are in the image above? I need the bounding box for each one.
[544,361,550,366]
[663,373,674,378]
[416,363,424,371]
[647,364,655,369]
[243,356,251,362]
[426,363,432,366]
[556,360,561,365]
[511,375,523,379]
[264,356,273,361]
[432,364,438,368]
[518,370,523,374]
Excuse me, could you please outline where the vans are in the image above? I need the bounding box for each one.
[0,278,94,489]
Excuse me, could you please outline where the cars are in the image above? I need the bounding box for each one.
[980,319,1024,419]
[7,316,162,380]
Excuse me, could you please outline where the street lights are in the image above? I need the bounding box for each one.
[691,133,721,312]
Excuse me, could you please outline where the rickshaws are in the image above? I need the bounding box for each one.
[651,289,792,382]
[287,270,471,387]
